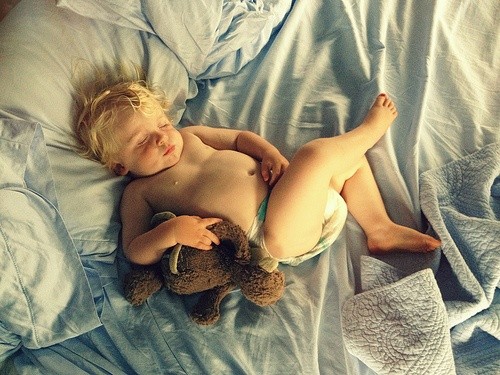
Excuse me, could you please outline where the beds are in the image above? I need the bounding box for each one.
[0,0,500,374]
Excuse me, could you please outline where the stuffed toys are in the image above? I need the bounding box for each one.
[123,212,286,325]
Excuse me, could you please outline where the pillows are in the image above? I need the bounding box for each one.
[51,0,293,91]
[0,0,198,260]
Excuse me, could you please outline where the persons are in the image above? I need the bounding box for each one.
[71,61,443,266]
